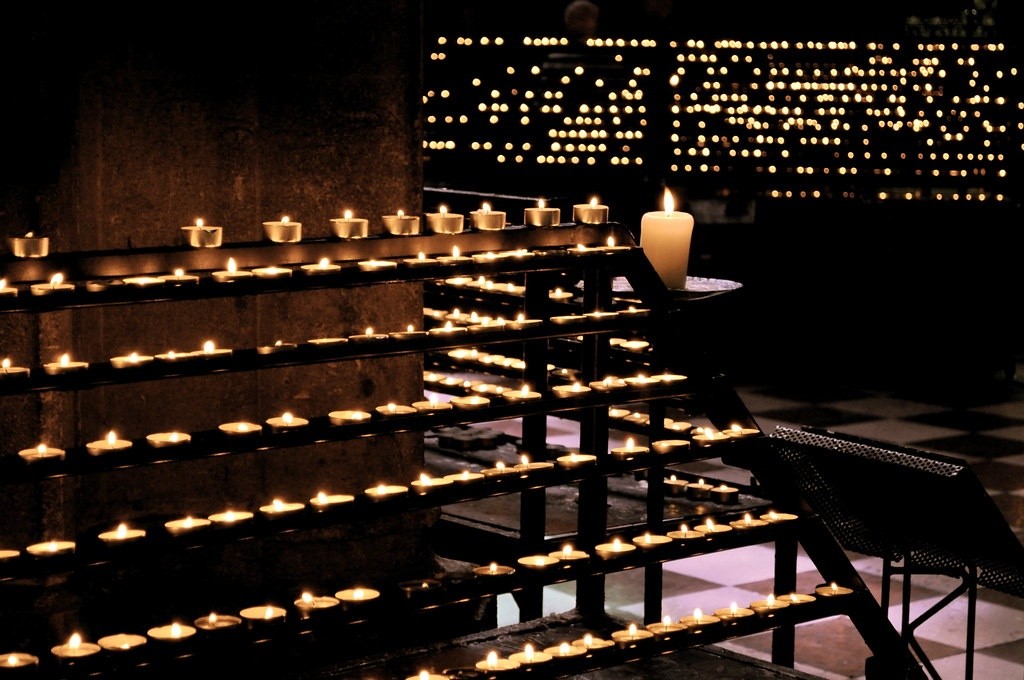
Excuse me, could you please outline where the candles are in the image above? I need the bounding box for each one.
[0,185,855,679]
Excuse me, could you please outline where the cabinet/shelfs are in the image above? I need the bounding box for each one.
[1,222,927,680]
[420,186,773,623]
